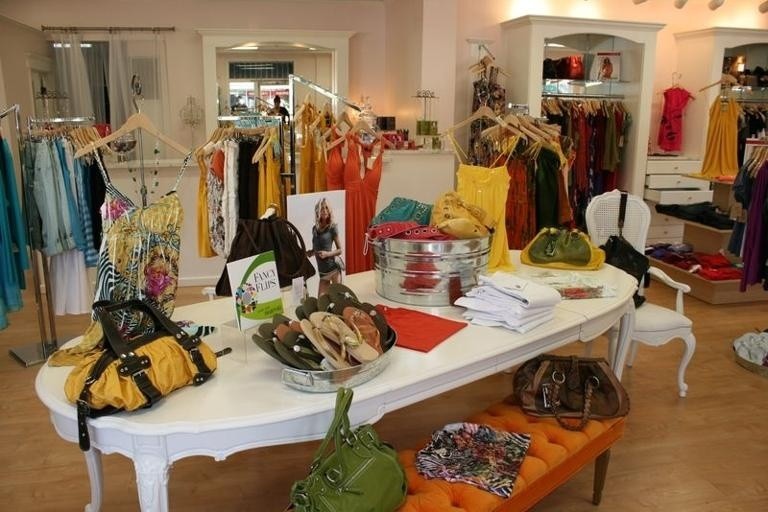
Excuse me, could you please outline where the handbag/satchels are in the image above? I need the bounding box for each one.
[520,228,605,271]
[598,235,650,307]
[513,355,630,429]
[64,299,218,418]
[290,387,408,511]
[544,56,583,79]
[215,213,316,295]
[369,194,494,243]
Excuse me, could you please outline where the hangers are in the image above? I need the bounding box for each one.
[470,53,512,79]
[697,68,754,95]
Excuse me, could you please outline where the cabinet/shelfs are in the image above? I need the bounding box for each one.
[499,14,667,201]
[644,154,715,246]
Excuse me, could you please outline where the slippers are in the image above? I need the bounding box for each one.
[252,282,385,372]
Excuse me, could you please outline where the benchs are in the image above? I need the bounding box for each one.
[395,391,628,512]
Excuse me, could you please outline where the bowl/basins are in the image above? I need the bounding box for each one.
[278,323,400,396]
[370,224,496,308]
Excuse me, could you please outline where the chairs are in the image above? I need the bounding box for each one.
[584,188,698,400]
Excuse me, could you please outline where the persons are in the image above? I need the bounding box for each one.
[303,196,345,298]
[601,57,614,80]
[267,95,290,123]
